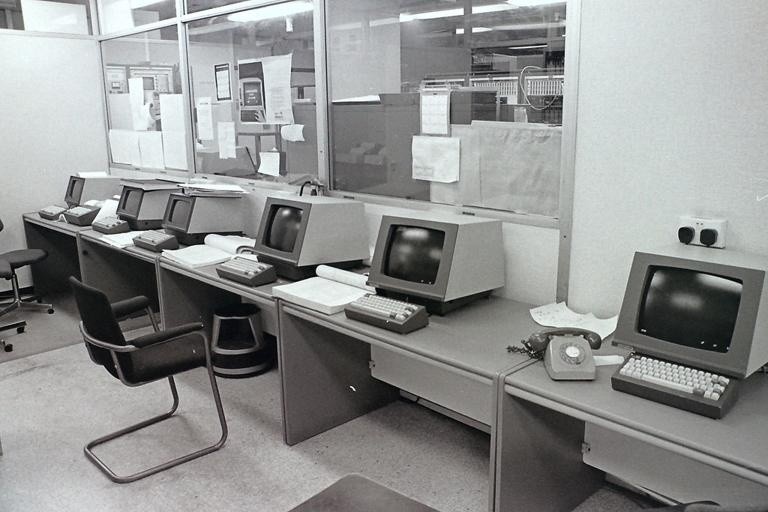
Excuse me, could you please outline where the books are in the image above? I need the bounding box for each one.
[271,265,377,316]
[162,233,257,269]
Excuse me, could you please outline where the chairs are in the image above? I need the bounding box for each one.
[69,276,226,483]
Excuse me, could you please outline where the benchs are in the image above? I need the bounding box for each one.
[0,221,55,352]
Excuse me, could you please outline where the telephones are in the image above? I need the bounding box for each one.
[530,328,601,380]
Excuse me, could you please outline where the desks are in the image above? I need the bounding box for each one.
[77,229,188,332]
[278,275,552,511]
[22,213,93,296]
[494,334,768,512]
[157,253,370,444]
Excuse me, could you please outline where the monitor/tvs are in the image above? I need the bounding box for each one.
[162,193,243,244]
[64,176,121,208]
[115,184,183,230]
[610,252,768,378]
[366,215,505,316]
[251,196,369,280]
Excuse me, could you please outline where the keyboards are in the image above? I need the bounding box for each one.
[216,256,277,287]
[344,293,429,334]
[609,352,739,419]
[92,217,131,234]
[63,206,101,226]
[133,230,179,252]
[39,205,67,220]
